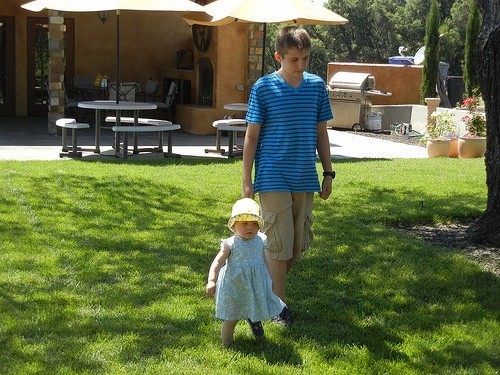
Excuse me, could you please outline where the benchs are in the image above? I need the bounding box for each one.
[205,118,247,158]
[56,116,181,159]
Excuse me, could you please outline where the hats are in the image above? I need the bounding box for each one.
[227,197,264,240]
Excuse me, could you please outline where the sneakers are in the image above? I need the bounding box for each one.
[270,306,293,326]
[247,319,264,336]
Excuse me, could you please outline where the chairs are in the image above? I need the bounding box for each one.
[44,74,179,159]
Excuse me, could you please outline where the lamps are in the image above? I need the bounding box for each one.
[98,11,108,24]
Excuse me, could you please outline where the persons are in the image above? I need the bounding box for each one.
[242,25,334,326]
[206,198,287,348]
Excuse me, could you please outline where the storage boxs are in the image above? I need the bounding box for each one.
[388,56,414,65]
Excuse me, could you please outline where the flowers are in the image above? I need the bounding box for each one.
[419,97,487,143]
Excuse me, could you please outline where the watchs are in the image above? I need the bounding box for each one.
[323,171,335,179]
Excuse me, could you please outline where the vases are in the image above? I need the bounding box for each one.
[427,137,487,158]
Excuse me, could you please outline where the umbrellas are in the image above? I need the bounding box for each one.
[183,0,349,78]
[20,0,214,104]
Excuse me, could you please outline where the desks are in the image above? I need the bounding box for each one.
[78,100,158,155]
[225,103,248,111]
[76,86,107,127]
